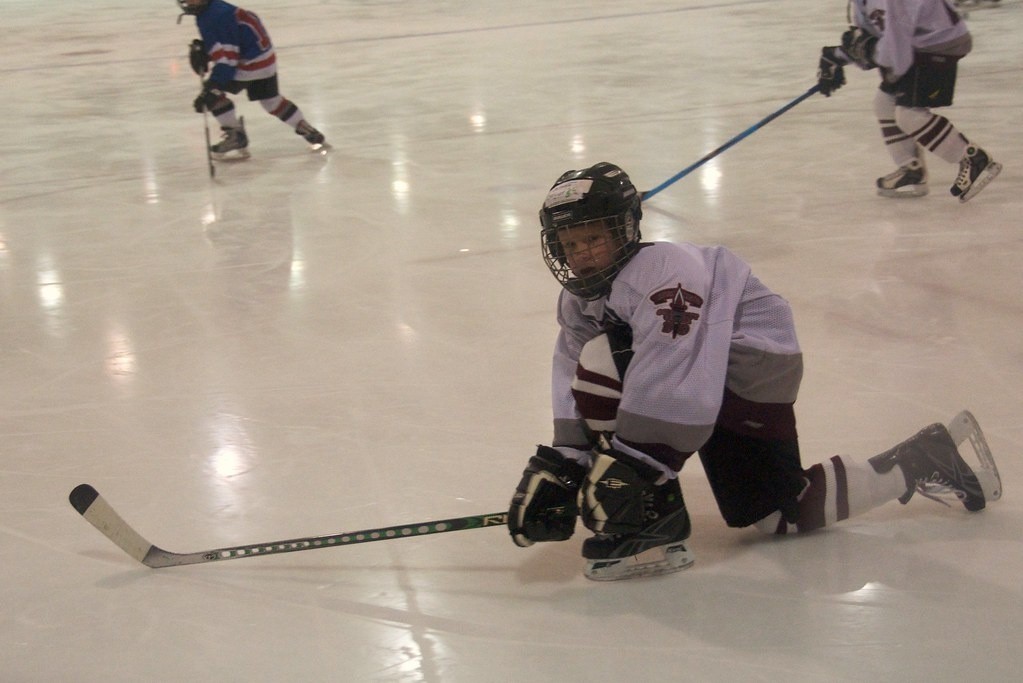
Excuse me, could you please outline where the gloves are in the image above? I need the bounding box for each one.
[577,467,644,534]
[815,47,844,95]
[508,444,587,548]
[189,38,208,74]
[193,87,219,112]
[840,25,877,69]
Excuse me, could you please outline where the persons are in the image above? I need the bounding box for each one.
[817,0,1002,204]
[179,0,331,162]
[508,162,1003,584]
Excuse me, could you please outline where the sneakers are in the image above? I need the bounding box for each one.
[950,143,1003,203]
[296,120,330,152]
[211,115,252,163]
[869,410,1004,512]
[876,162,930,196]
[583,472,693,582]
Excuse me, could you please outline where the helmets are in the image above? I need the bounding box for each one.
[178,0,209,17]
[538,161,643,302]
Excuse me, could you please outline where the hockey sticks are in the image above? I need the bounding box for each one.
[66,479,584,572]
[635,73,844,203]
[194,44,231,190]
